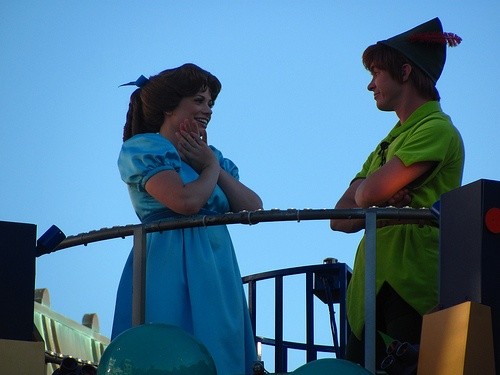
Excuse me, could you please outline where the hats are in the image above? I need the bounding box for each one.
[377,16,464,85]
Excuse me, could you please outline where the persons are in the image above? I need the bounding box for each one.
[110,63,257,375]
[331,17,466,375]
